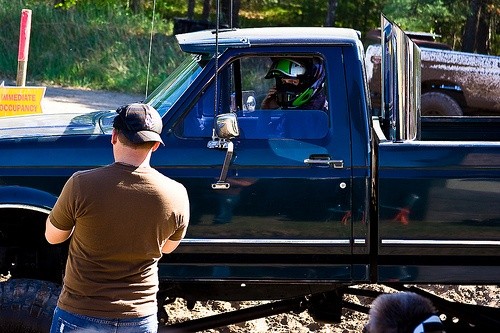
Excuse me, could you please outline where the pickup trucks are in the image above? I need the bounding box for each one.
[0,12,500,333]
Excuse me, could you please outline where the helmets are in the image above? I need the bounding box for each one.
[265,56,326,110]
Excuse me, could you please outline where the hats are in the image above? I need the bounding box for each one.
[113,102,165,146]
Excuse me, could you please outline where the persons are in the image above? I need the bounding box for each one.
[367,291,443,333]
[260,57,329,114]
[44,103,190,333]
[189,177,258,225]
